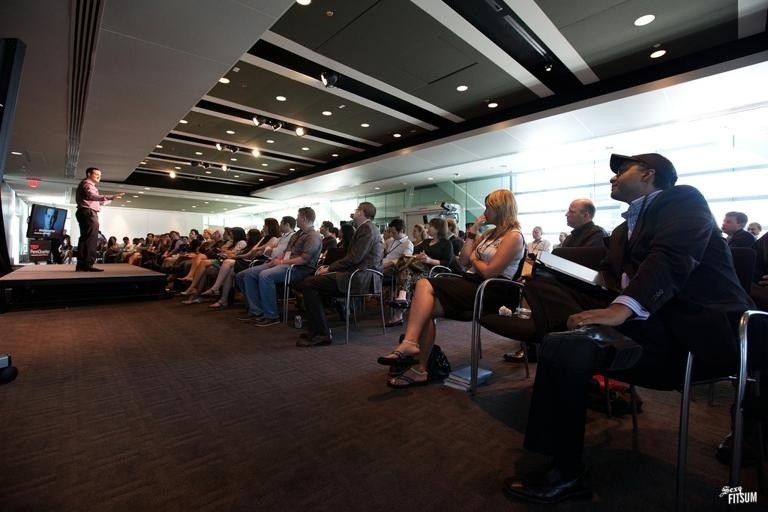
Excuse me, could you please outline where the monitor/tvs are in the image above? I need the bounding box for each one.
[27,204,68,240]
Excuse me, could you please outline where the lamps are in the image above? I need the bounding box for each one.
[230,147,239,153]
[253,116,268,127]
[190,161,199,166]
[270,121,283,132]
[296,127,309,137]
[216,144,226,151]
[321,70,342,87]
[202,162,209,169]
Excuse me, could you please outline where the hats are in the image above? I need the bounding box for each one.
[610,153,678,182]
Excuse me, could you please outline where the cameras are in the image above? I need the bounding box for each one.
[440,202,457,212]
[528,253,536,259]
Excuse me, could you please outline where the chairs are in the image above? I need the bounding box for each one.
[429,248,527,395]
[474,251,539,393]
[395,264,452,299]
[250,242,323,323]
[730,311,768,512]
[592,249,753,512]
[326,269,387,344]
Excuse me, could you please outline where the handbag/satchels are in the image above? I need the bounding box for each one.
[399,335,450,380]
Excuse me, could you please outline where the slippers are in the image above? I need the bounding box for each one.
[377,351,420,366]
[390,369,430,388]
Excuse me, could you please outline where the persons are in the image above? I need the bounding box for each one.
[74,167,126,272]
[553,231,568,253]
[504,151,759,504]
[385,217,454,328]
[41,207,59,240]
[410,222,430,256]
[133,233,170,257]
[235,207,323,328]
[525,226,552,258]
[382,223,394,256]
[170,228,214,293]
[164,229,185,276]
[443,218,464,259]
[330,227,341,244]
[119,237,133,264]
[177,226,232,297]
[379,219,415,286]
[317,221,339,263]
[377,189,526,389]
[182,228,202,260]
[96,229,107,250]
[210,228,224,253]
[208,228,261,301]
[505,198,613,365]
[57,238,72,264]
[336,224,356,250]
[237,216,297,317]
[61,229,72,240]
[180,227,247,305]
[199,218,283,310]
[105,236,120,264]
[748,222,765,241]
[721,211,767,290]
[296,201,385,348]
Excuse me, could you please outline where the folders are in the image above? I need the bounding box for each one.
[531,248,607,289]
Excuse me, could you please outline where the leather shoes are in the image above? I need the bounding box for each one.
[296,329,333,346]
[386,299,409,308]
[85,266,105,272]
[385,320,404,325]
[509,466,594,505]
[504,345,538,362]
[75,266,84,270]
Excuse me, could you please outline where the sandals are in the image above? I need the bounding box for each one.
[201,288,220,296]
[208,301,229,310]
[182,298,201,304]
[180,290,198,295]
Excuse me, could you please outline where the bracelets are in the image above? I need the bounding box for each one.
[467,230,477,242]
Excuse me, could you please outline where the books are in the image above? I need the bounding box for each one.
[442,381,476,392]
[448,364,494,384]
[445,375,489,389]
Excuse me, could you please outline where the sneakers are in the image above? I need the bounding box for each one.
[254,317,281,326]
[239,313,254,320]
